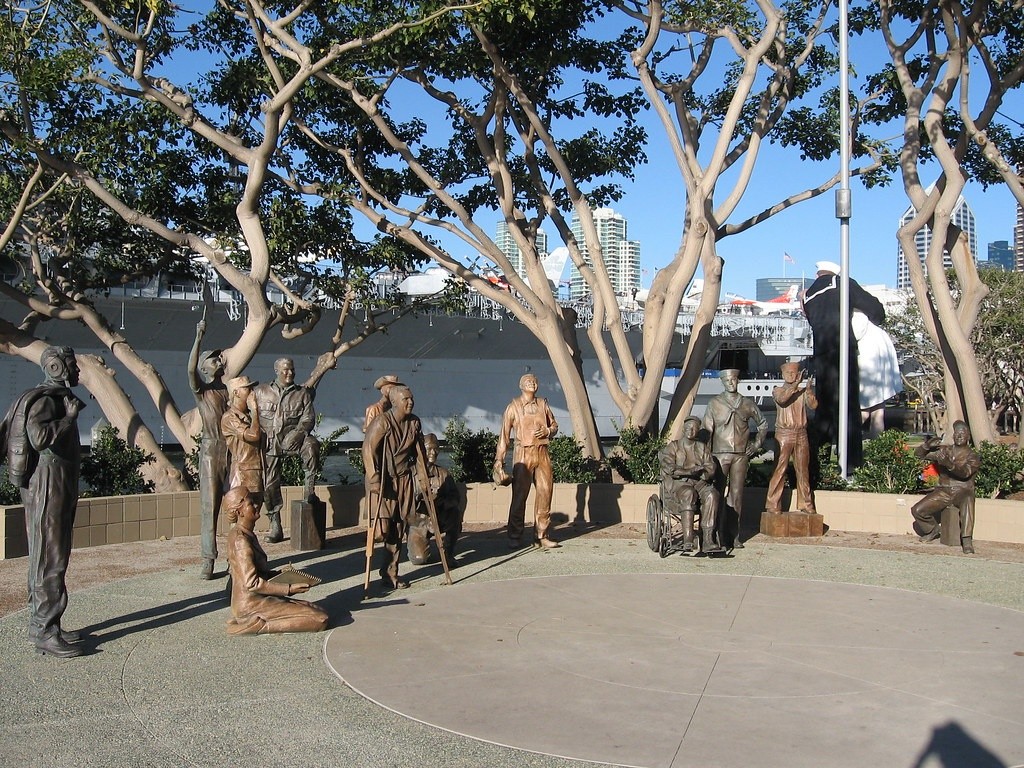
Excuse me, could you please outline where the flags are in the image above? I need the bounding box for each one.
[785,252,797,264]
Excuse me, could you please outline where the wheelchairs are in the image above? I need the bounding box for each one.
[645,474,727,558]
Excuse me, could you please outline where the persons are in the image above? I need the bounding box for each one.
[187,320,453,636]
[803,260,886,476]
[0,345,88,658]
[494,375,559,548]
[661,417,722,550]
[695,370,769,549]
[765,363,817,514]
[848,307,905,439]
[909,420,980,554]
